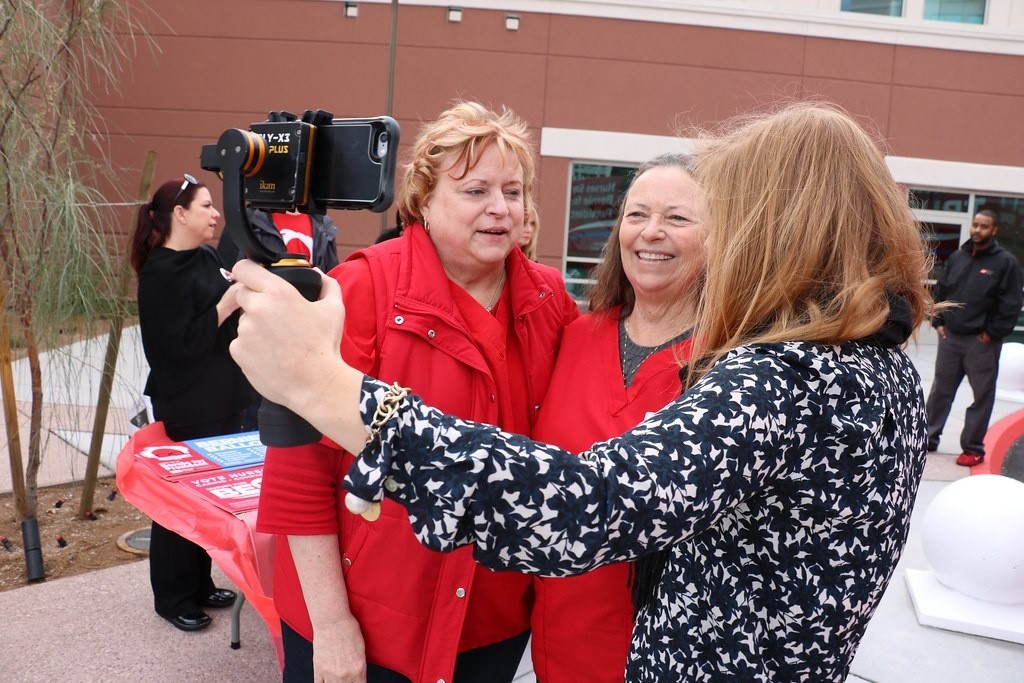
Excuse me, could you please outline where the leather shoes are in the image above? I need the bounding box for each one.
[197,588,237,606]
[157,606,212,629]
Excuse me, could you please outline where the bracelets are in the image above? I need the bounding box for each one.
[346,382,413,522]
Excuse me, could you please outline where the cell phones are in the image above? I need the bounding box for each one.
[306,114,400,213]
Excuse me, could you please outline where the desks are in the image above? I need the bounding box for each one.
[114,422,286,680]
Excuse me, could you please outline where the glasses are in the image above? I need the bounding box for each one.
[172,173,198,203]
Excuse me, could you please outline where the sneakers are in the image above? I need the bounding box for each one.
[928,446,936,451]
[956,451,984,465]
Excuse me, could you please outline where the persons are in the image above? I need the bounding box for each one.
[376,209,405,245]
[254,99,581,683]
[529,159,712,683]
[518,204,540,262]
[216,207,339,275]
[924,209,1024,466]
[127,176,243,630]
[230,106,935,683]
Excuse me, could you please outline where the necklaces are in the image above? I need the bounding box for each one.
[622,314,695,383]
[487,272,504,314]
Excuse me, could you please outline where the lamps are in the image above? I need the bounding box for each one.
[448,6,462,21]
[504,16,519,30]
[343,2,359,17]
